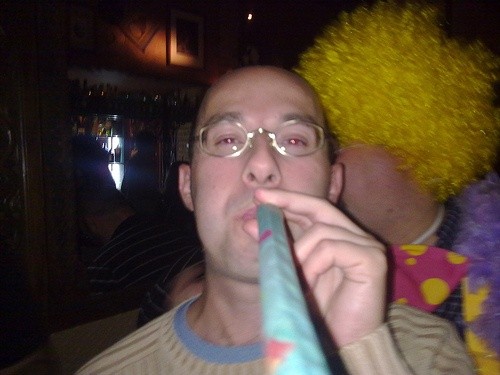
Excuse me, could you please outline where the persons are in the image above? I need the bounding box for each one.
[285,8,498,375]
[72,64,479,374]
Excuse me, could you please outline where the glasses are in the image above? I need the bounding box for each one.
[187,118,338,161]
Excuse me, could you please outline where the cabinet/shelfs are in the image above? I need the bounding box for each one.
[74,114,127,164]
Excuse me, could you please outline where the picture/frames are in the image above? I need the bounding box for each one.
[166,5,207,71]
